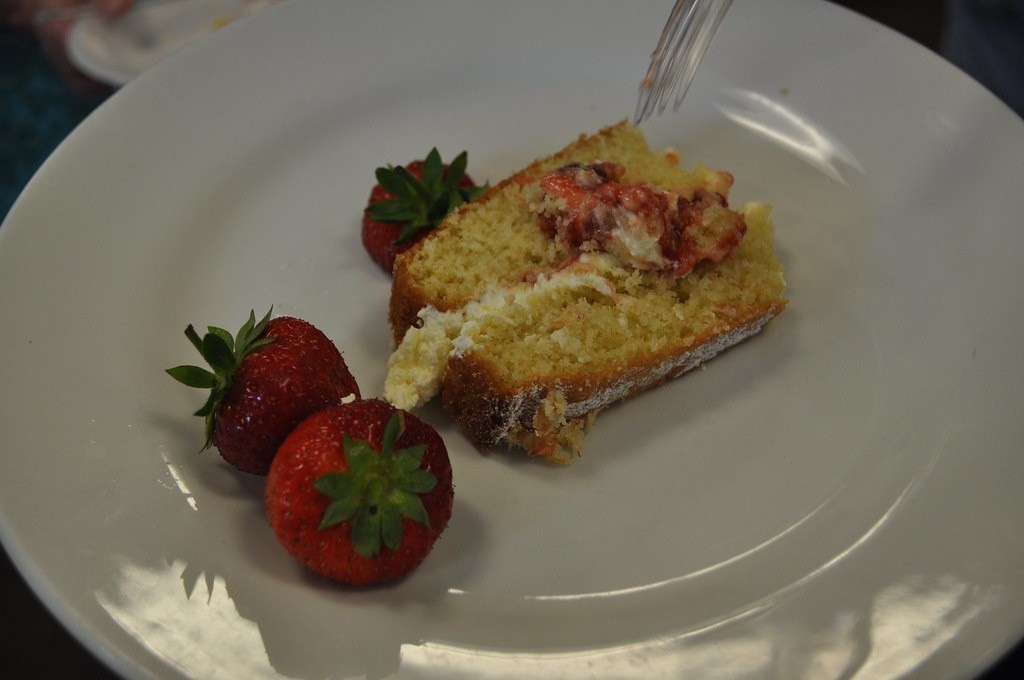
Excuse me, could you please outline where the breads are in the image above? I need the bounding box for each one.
[388,116,786,462]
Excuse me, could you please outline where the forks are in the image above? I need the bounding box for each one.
[632,0,733,127]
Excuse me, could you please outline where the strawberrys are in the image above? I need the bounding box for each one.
[164,305,360,475]
[362,149,489,278]
[266,398,452,583]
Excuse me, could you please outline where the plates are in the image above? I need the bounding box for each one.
[65,1,269,88]
[0,0,1024,680]
